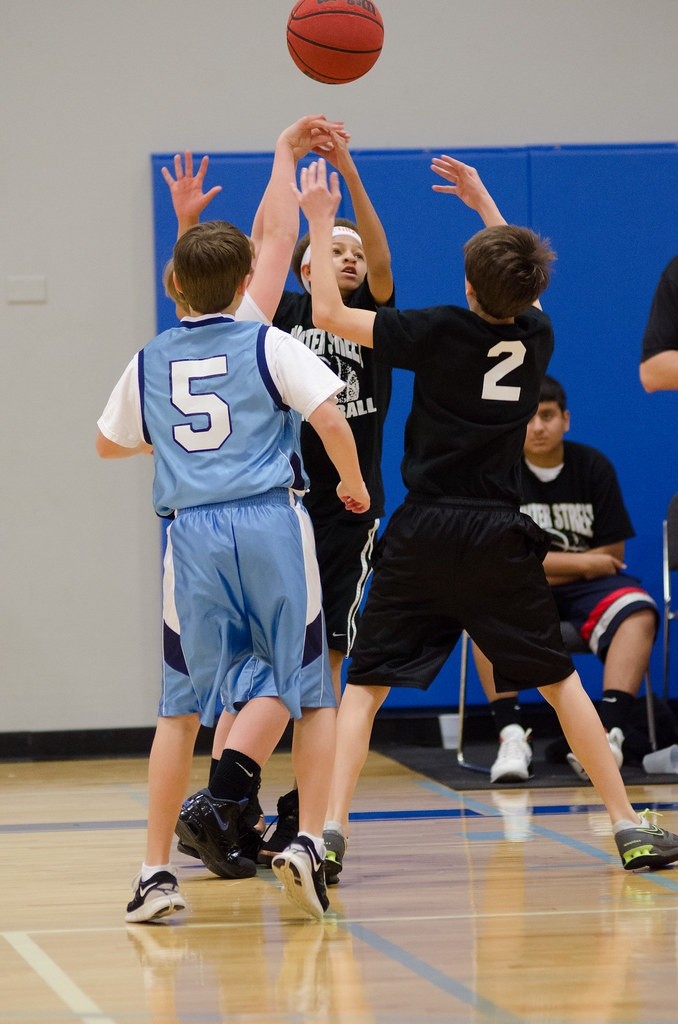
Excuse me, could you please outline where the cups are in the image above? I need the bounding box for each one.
[438,713,461,750]
[642,744,678,775]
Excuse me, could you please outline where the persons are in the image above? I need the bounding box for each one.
[161,115,396,878]
[95,220,371,922]
[639,255,678,393]
[290,156,678,884]
[472,374,661,783]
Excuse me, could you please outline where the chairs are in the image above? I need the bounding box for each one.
[456,622,658,774]
[662,494,678,717]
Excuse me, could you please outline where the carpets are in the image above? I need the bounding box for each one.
[370,740,678,791]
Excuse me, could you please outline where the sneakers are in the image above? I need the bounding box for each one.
[566,727,624,781]
[489,724,532,781]
[271,833,331,920]
[175,787,258,879]
[256,787,300,870]
[322,833,344,884]
[124,866,186,921]
[615,808,678,872]
[177,824,268,865]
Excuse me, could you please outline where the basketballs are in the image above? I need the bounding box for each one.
[285,0,385,86]
